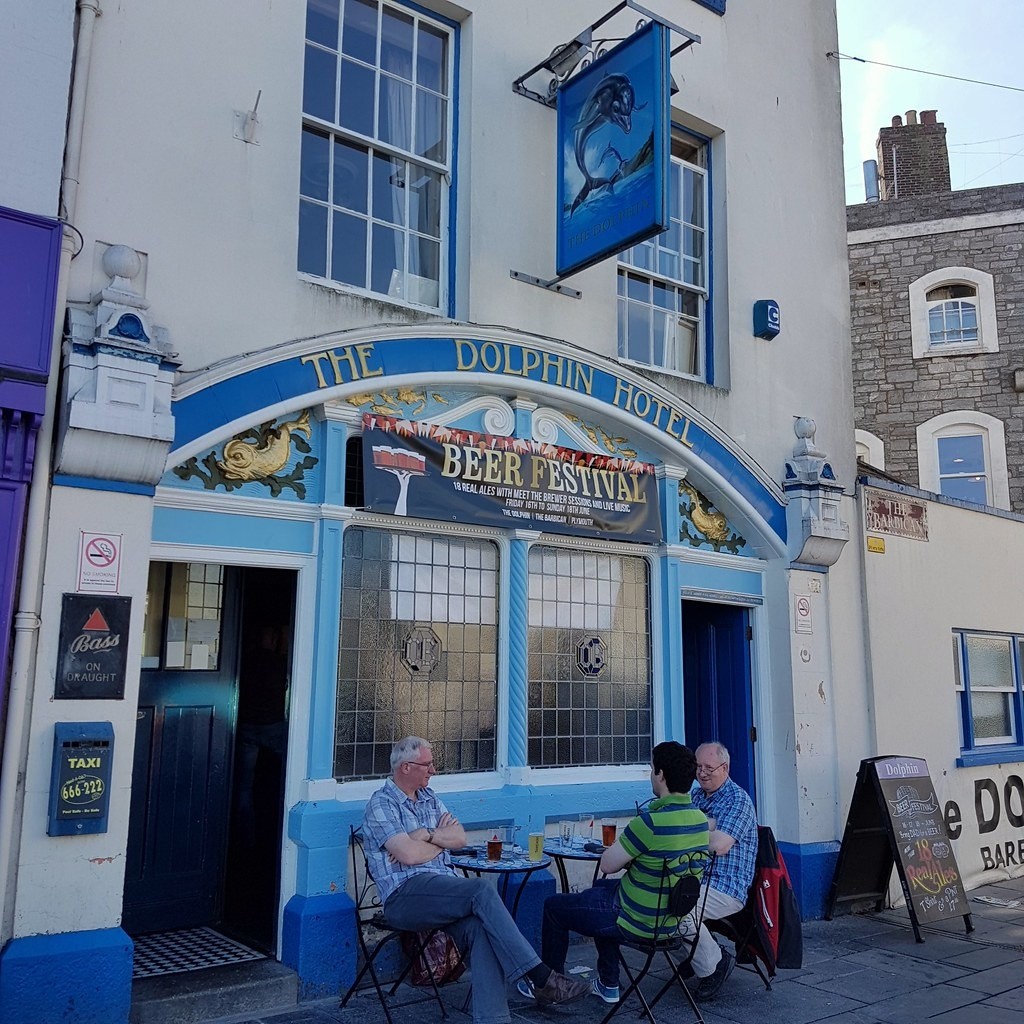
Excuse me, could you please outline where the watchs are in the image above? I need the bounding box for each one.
[427,828,435,843]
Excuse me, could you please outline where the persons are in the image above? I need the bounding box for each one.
[666,741,758,1000]
[517,742,710,1003]
[362,735,594,1024]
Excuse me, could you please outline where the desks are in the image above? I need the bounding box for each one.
[437,845,552,1012]
[543,838,624,990]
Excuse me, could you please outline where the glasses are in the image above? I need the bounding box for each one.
[407,761,433,769]
[697,762,726,775]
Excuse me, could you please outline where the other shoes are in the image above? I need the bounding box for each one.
[695,948,736,1001]
[677,960,696,980]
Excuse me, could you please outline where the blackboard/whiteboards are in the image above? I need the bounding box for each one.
[827,753,972,927]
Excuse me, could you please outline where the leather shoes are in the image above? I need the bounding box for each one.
[533,969,594,1005]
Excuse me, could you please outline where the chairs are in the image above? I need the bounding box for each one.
[340,824,448,1024]
[704,826,773,998]
[601,849,716,1024]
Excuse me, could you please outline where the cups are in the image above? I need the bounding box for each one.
[529,833,544,863]
[579,814,593,841]
[499,825,514,859]
[601,818,617,847]
[559,821,575,852]
[488,829,504,861]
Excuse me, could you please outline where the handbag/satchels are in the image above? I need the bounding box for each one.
[410,929,466,986]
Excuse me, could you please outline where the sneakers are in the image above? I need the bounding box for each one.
[589,977,619,1003]
[518,980,534,998]
